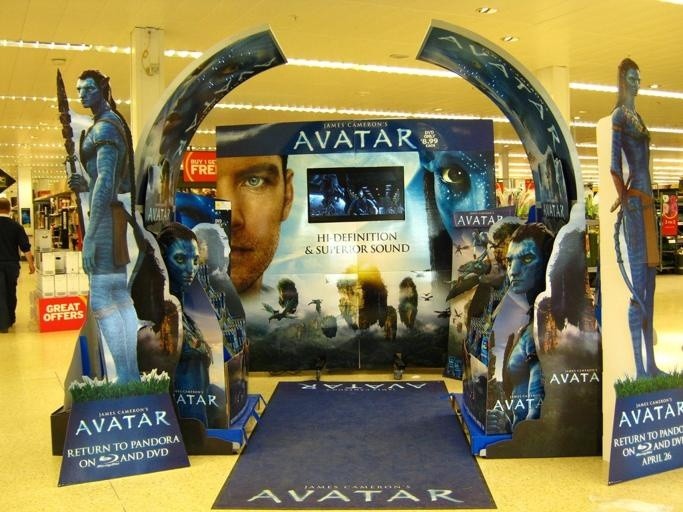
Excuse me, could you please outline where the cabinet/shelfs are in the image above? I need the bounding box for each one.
[651,184,683,275]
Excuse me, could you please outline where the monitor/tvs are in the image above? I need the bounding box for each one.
[307,166,406,223]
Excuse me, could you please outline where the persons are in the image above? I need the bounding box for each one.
[63,68,147,388]
[157,220,212,431]
[431,151,497,249]
[607,57,671,379]
[0,196,34,332]
[501,222,558,431]
[215,122,300,298]
